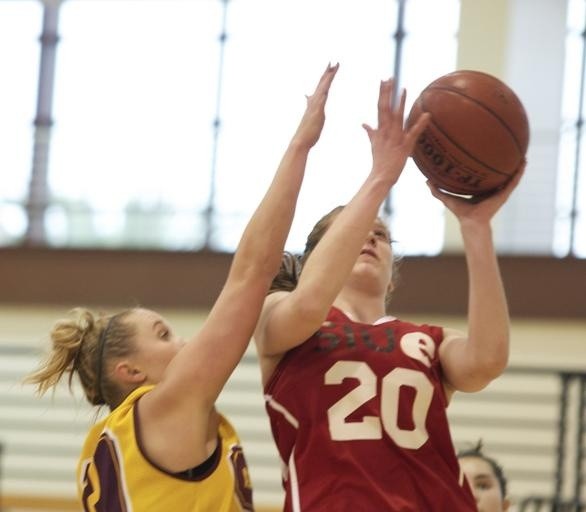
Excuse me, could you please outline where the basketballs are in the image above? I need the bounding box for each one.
[405,70,529,196]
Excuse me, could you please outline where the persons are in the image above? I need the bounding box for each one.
[451,433,520,512]
[16,57,342,510]
[224,67,531,510]
